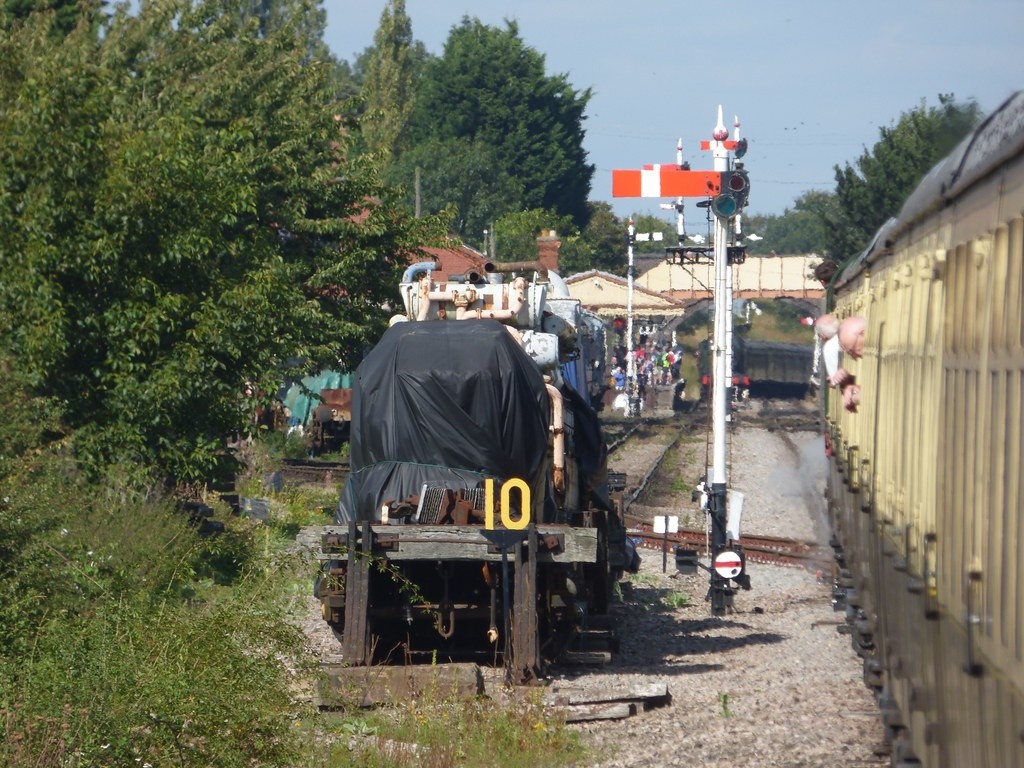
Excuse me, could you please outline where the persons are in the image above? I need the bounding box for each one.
[816,314,843,376]
[610,318,682,410]
[837,314,867,411]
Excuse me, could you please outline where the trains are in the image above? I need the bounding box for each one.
[696,327,816,403]
[819,89,1024,768]
[308,265,642,683]
